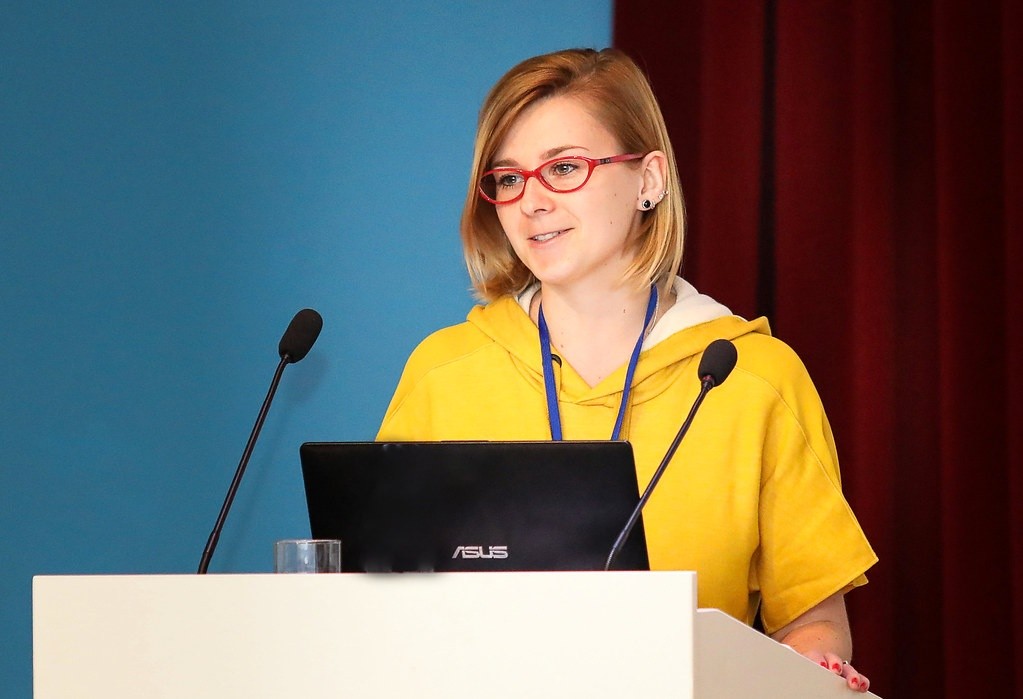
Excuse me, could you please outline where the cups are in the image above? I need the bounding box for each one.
[273,539,341,574]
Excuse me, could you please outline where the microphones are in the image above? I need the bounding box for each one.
[198,309,324,574]
[606,338,737,573]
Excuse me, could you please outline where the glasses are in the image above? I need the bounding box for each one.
[478,151,646,205]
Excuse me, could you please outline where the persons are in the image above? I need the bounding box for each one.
[371,45,878,697]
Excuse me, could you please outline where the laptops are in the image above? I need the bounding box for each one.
[299,440,651,573]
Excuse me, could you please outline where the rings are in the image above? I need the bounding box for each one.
[843,661,851,664]
